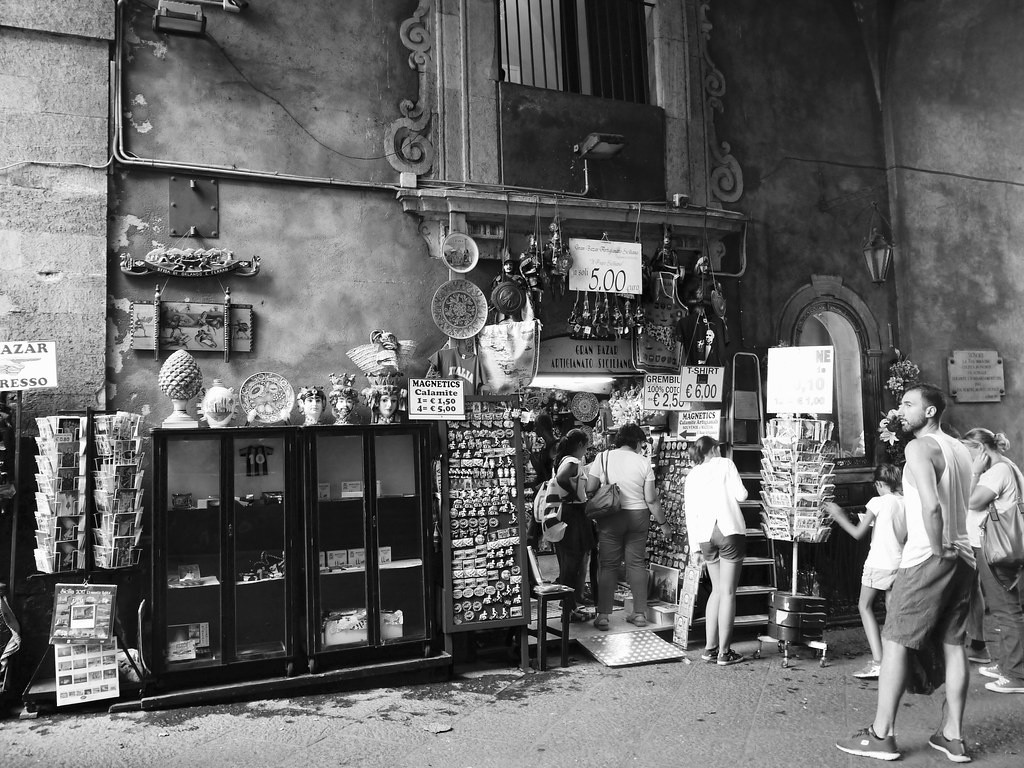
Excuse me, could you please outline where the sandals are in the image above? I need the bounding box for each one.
[626,613,647,626]
[561,612,591,622]
[577,609,596,617]
[594,616,609,630]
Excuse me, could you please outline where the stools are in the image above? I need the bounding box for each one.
[527,545,574,668]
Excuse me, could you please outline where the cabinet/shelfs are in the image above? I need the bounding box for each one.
[152,427,429,666]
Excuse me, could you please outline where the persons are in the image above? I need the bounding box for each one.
[936,422,1024,693]
[834,383,975,763]
[587,424,674,630]
[329,372,359,425]
[697,329,721,365]
[491,248,523,323]
[551,428,597,620]
[362,374,404,425]
[684,437,745,665]
[298,385,327,427]
[825,463,905,675]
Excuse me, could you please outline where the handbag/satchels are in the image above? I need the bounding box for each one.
[534,455,566,523]
[906,631,946,695]
[584,449,621,520]
[978,461,1024,565]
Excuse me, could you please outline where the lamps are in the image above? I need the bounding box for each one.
[579,133,627,160]
[151,0,208,36]
[862,201,897,288]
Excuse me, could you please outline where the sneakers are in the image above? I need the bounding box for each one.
[929,734,973,762]
[985,675,1024,693]
[702,645,719,662]
[852,660,881,678]
[717,649,744,665]
[979,664,1003,678]
[836,725,901,760]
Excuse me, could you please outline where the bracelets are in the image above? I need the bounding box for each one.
[658,519,667,526]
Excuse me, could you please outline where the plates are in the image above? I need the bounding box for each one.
[442,232,479,273]
[239,372,295,424]
[430,278,489,340]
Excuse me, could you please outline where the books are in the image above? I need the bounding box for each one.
[35,413,86,574]
[760,420,834,543]
[95,410,143,565]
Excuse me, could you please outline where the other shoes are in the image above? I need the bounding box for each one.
[558,601,585,609]
[581,599,594,607]
[967,647,991,663]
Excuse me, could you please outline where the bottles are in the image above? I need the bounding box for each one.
[172,493,192,507]
[195,379,238,426]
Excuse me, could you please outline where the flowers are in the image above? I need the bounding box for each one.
[879,347,919,463]
